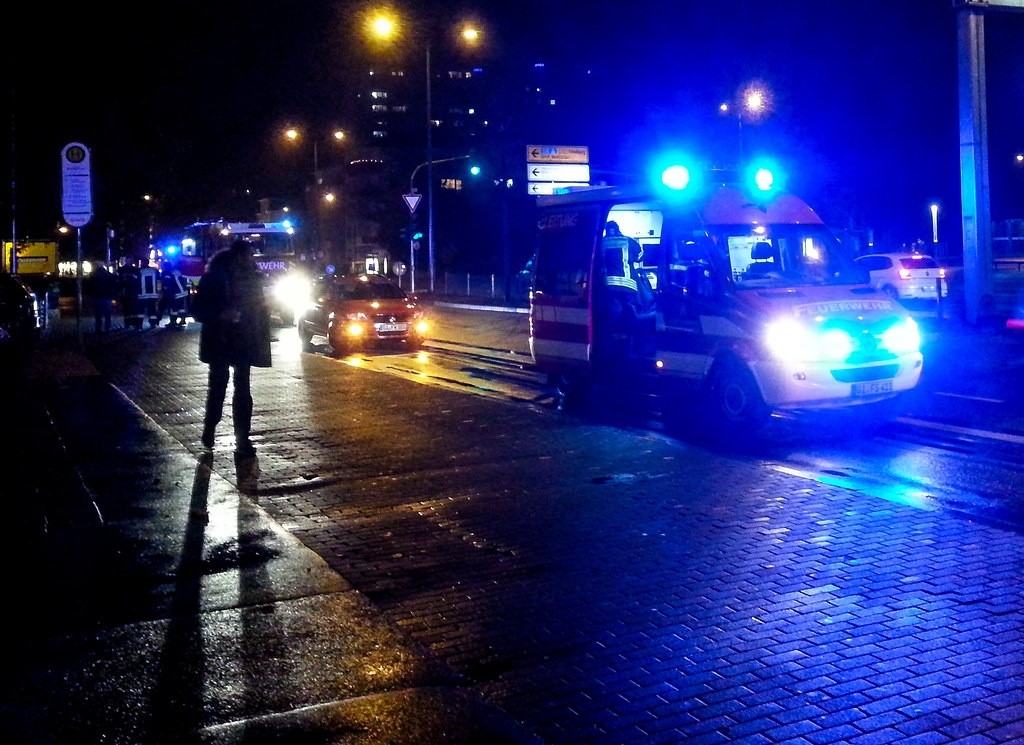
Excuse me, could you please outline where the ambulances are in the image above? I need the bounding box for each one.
[530,177,925,439]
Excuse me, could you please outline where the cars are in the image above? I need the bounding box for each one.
[298,272,428,353]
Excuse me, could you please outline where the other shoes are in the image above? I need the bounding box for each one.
[236,435,249,449]
[201,427,214,446]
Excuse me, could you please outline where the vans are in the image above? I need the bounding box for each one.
[854,253,948,305]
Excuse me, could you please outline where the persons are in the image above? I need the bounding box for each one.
[596,219,643,407]
[193,238,273,457]
[911,236,932,257]
[82,256,192,330]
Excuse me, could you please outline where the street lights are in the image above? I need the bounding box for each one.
[408,154,480,292]
[360,4,480,293]
[280,125,345,270]
[56,214,82,345]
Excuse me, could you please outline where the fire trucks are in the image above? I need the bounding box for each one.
[178,220,298,326]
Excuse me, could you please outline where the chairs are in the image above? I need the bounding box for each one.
[679,240,711,292]
[746,242,778,279]
